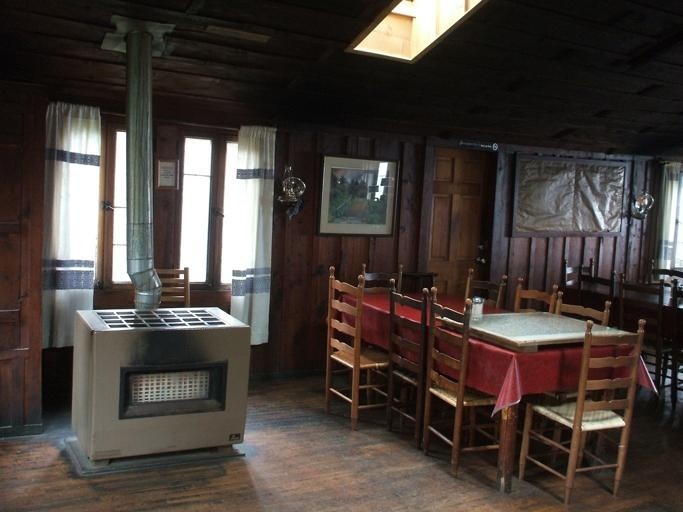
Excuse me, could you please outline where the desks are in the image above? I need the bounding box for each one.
[340,286,662,493]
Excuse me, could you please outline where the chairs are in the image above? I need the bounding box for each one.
[555,254,683,414]
[154,265,190,306]
[517,314,646,509]
[421,287,503,480]
[386,278,428,451]
[362,261,612,399]
[320,265,388,424]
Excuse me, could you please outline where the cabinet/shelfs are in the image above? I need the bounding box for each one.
[318,154,398,236]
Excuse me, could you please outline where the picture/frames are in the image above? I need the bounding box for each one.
[510,152,626,238]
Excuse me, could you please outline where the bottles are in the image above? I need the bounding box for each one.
[469,296,486,320]
